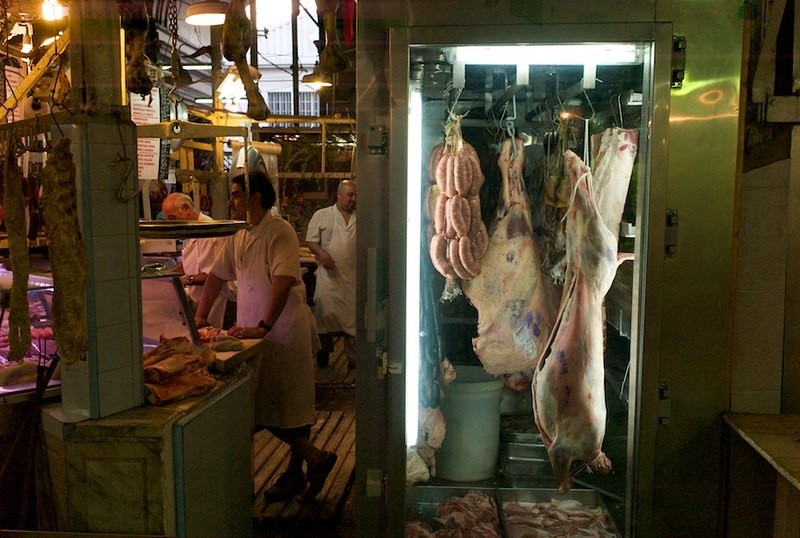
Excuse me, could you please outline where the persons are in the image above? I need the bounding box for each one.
[304,180,357,368]
[194,170,338,501]
[161,193,229,329]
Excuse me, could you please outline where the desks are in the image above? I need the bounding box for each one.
[720,408,800,538]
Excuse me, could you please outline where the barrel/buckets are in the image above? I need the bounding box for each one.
[434,365,505,480]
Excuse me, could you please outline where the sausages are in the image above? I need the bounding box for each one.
[425,140,489,280]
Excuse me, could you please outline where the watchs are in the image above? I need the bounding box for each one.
[258,320,272,332]
[186,275,195,286]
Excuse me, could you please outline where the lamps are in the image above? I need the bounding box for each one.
[301,60,332,85]
[184,0,231,27]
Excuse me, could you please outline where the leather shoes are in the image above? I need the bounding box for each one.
[262,470,306,500]
[300,449,338,501]
